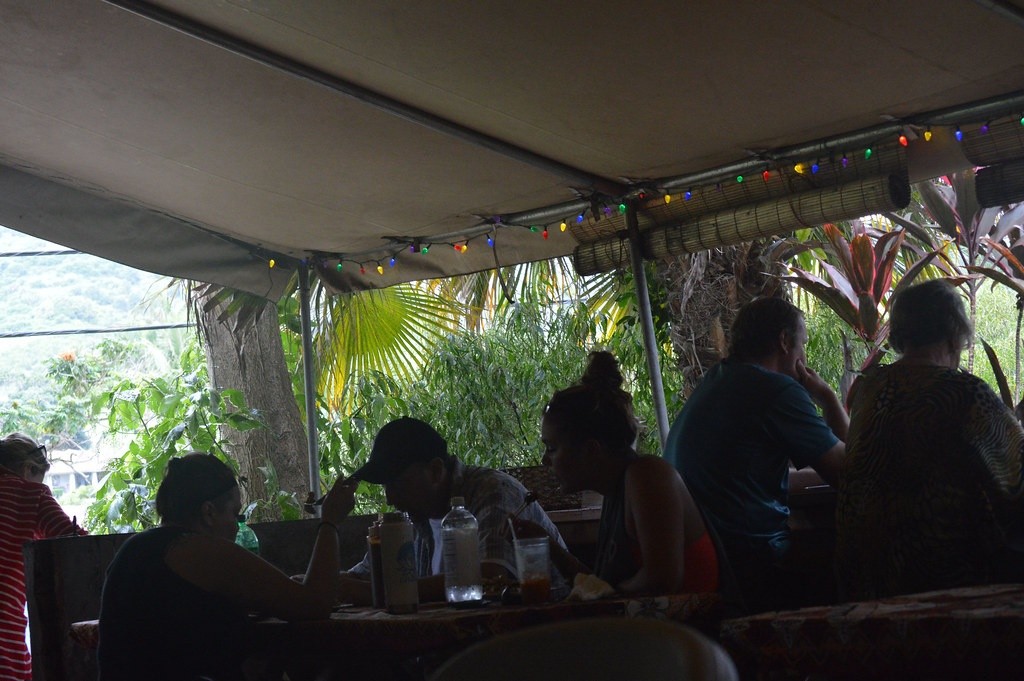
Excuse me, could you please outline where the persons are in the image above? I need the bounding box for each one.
[499,351,718,594]
[659,297,857,613]
[289,415,573,610]
[97,454,359,681]
[833,276,1024,602]
[0,434,90,681]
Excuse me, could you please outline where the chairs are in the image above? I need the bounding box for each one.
[423,616,740,681]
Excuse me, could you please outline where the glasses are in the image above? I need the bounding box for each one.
[17,443,48,465]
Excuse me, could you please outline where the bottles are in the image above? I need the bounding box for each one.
[442,496,482,602]
[235,515,259,557]
[367,521,384,607]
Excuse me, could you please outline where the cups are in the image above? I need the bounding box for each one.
[513,537,550,603]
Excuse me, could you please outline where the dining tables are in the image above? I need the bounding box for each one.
[70,597,628,648]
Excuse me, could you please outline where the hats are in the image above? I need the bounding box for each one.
[354,416,445,483]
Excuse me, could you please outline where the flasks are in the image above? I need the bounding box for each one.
[379,511,419,613]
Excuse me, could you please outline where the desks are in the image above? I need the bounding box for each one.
[721,583,1024,681]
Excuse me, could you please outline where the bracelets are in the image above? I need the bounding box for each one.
[317,517,340,537]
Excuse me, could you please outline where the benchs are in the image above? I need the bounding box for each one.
[22,513,378,681]
